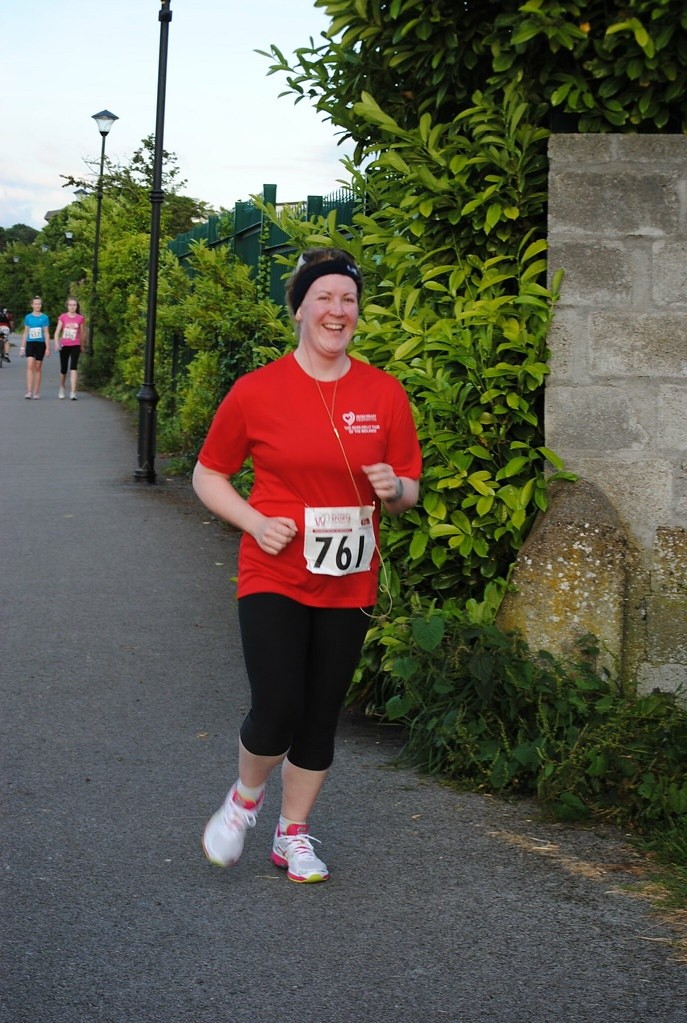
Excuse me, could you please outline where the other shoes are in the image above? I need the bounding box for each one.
[4,353,11,362]
[33,393,40,400]
[25,393,31,399]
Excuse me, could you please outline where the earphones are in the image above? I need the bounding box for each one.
[297,307,301,319]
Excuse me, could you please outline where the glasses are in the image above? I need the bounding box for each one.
[295,249,354,273]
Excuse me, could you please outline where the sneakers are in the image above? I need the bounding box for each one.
[269,824,329,883]
[202,779,266,868]
[69,392,78,400]
[59,389,65,399]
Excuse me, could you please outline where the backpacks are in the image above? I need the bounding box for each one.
[0,307,9,323]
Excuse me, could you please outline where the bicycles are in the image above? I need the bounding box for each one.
[0,329,13,368]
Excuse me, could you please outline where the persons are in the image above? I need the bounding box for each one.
[0,308,15,363]
[191,248,421,882]
[20,296,50,400]
[54,297,85,400]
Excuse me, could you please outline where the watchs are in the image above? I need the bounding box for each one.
[386,477,403,503]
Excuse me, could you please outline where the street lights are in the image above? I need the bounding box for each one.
[86,108,119,368]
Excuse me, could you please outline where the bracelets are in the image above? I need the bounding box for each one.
[21,347,26,349]
